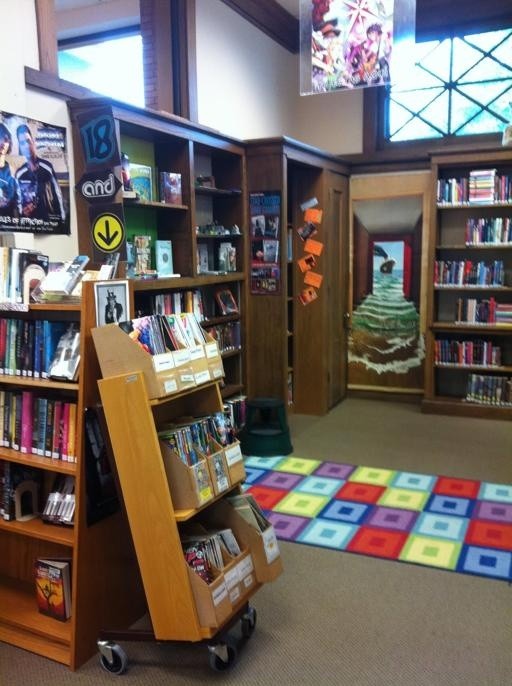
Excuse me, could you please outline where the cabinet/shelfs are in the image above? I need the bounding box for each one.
[423,143,512,420]
[249,135,351,415]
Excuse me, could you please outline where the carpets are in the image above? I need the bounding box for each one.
[240,453,511,581]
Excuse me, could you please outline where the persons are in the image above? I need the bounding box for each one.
[0,123,20,223]
[103,286,119,322]
[17,122,65,221]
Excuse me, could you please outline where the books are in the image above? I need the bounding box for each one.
[123,157,240,395]
[2,247,119,625]
[120,314,271,584]
[432,168,511,402]
